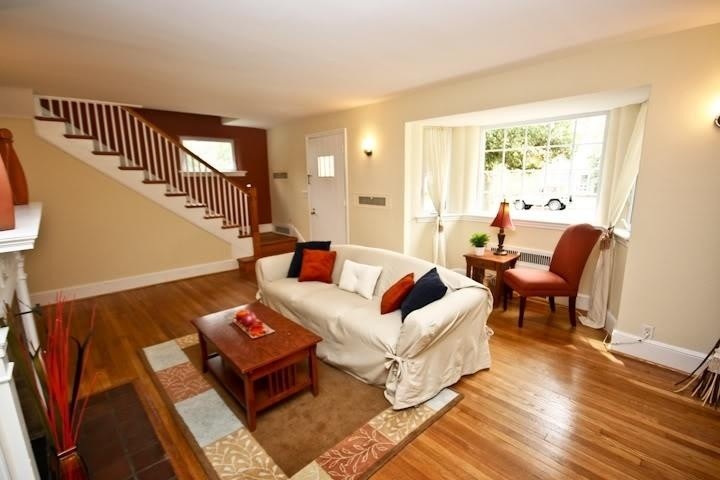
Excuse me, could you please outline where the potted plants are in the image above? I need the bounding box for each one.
[469,231,490,256]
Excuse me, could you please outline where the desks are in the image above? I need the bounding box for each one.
[462,253,521,308]
[191,301,323,432]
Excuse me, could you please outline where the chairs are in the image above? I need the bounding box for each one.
[503,224,603,328]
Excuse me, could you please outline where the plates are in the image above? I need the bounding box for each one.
[232,317,275,339]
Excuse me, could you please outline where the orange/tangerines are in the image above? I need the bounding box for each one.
[235,310,247,320]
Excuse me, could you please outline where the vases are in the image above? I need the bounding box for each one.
[49,445,90,480]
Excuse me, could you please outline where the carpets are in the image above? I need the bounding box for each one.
[135,331,464,480]
[68,382,178,480]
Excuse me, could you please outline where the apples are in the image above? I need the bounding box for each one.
[242,312,256,325]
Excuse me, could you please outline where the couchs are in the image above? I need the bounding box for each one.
[254,245,495,410]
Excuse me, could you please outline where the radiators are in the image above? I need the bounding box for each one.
[489,244,555,269]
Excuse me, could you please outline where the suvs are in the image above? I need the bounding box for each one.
[502,183,575,212]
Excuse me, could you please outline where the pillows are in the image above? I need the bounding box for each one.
[401,267,448,324]
[298,247,336,283]
[381,272,414,316]
[339,259,382,300]
[287,241,331,278]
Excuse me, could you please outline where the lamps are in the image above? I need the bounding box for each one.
[362,138,371,156]
[715,112,719,129]
[489,199,516,256]
